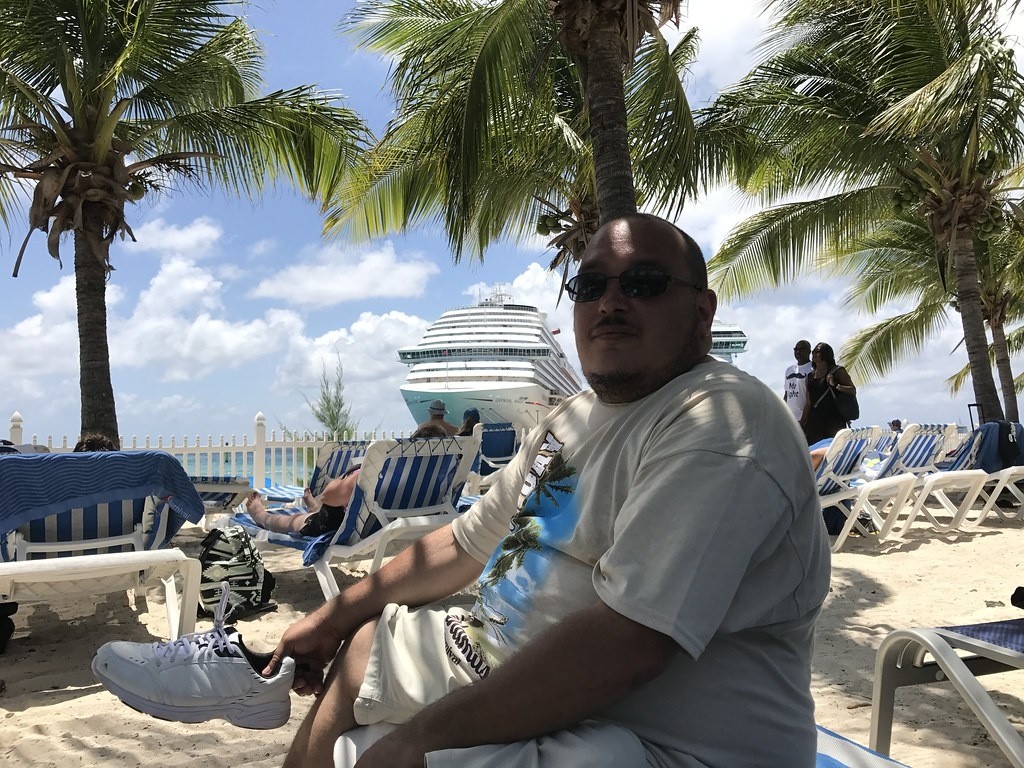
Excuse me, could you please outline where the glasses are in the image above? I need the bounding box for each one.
[565,268,704,300]
[811,350,822,354]
[794,348,808,352]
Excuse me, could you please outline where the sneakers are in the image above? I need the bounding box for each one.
[91,626,294,732]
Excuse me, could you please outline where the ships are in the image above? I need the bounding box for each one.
[397,282,580,454]
[707,319,751,368]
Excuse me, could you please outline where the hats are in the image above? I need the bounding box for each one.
[463,408,479,422]
[427,399,449,414]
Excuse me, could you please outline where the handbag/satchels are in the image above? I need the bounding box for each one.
[828,365,859,420]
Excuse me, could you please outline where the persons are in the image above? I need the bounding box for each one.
[246,423,447,537]
[798,342,859,445]
[783,340,814,424]
[417,398,460,437]
[263,212,833,767]
[887,419,903,452]
[454,408,481,437]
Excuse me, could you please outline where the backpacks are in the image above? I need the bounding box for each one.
[199,525,276,614]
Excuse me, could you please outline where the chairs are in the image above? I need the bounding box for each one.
[1,419,1024,767]
